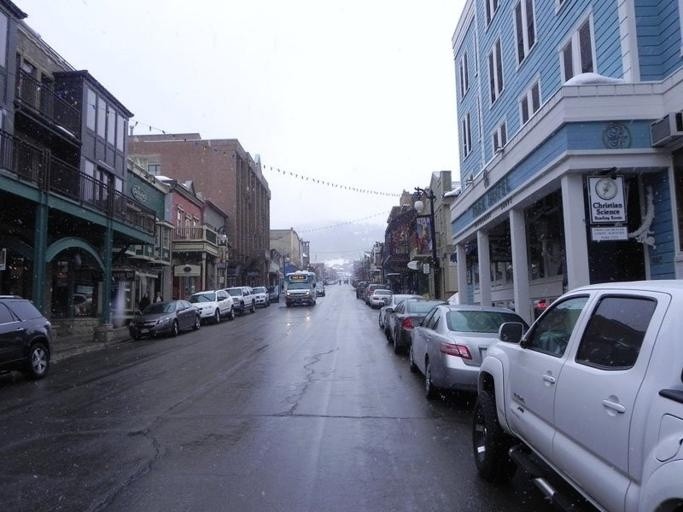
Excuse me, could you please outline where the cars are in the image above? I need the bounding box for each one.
[343,275,450,355]
[315,276,338,298]
[185,282,280,323]
[126,298,203,341]
[407,302,535,402]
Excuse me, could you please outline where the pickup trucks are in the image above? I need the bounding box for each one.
[469,277,683,511]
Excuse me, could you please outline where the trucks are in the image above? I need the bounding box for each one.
[282,269,317,308]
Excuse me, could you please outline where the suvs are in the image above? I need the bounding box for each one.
[0,293,55,381]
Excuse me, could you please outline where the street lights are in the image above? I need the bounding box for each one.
[413,185,442,300]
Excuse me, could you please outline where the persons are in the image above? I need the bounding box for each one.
[138,293,151,312]
[153,291,163,303]
